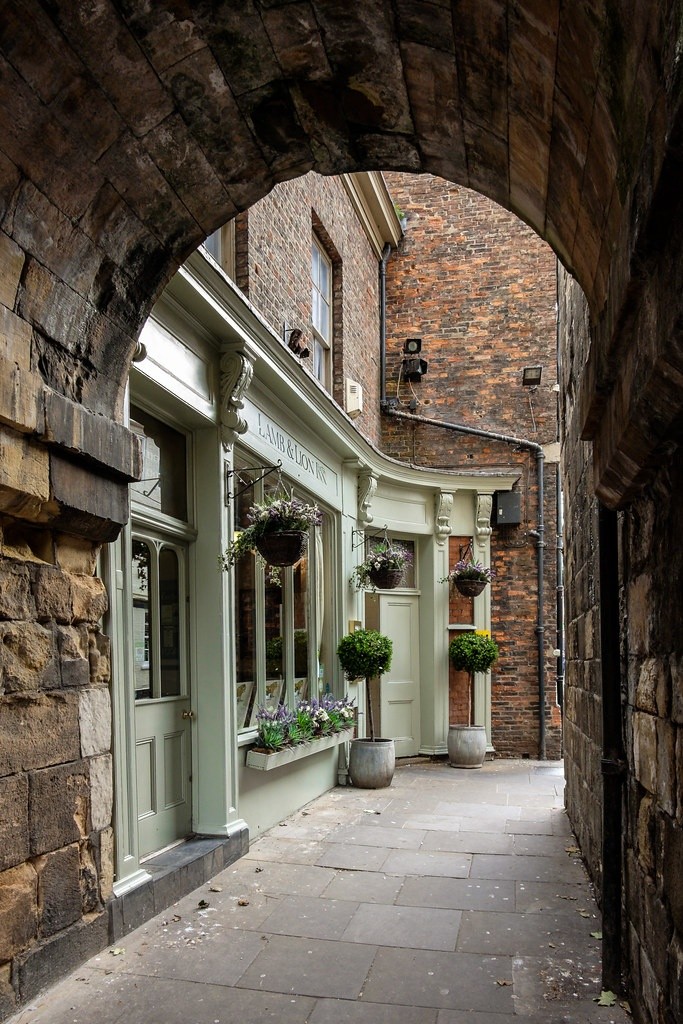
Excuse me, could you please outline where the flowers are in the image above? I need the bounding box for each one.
[256,693,357,750]
[217,489,325,588]
[437,559,496,600]
[348,542,413,600]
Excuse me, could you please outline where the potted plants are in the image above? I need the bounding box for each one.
[337,629,395,789]
[447,632,498,768]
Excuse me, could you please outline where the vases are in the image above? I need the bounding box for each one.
[246,727,355,771]
[369,569,403,590]
[256,530,309,566]
[455,580,487,597]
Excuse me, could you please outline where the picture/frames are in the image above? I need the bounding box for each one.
[349,621,364,683]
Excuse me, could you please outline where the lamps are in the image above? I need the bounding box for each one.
[403,339,421,354]
[523,367,542,386]
[405,358,427,376]
